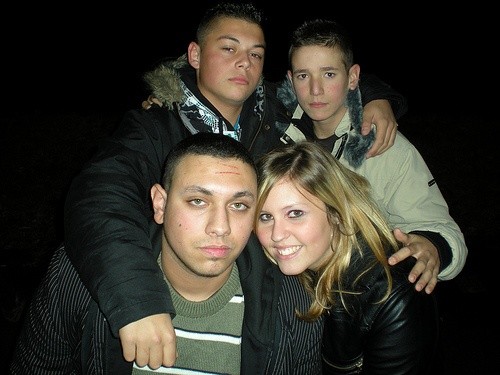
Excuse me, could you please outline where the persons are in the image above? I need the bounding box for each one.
[252,140,417,375]
[74,0,398,370]
[7,133,323,375]
[272,18,470,295]
